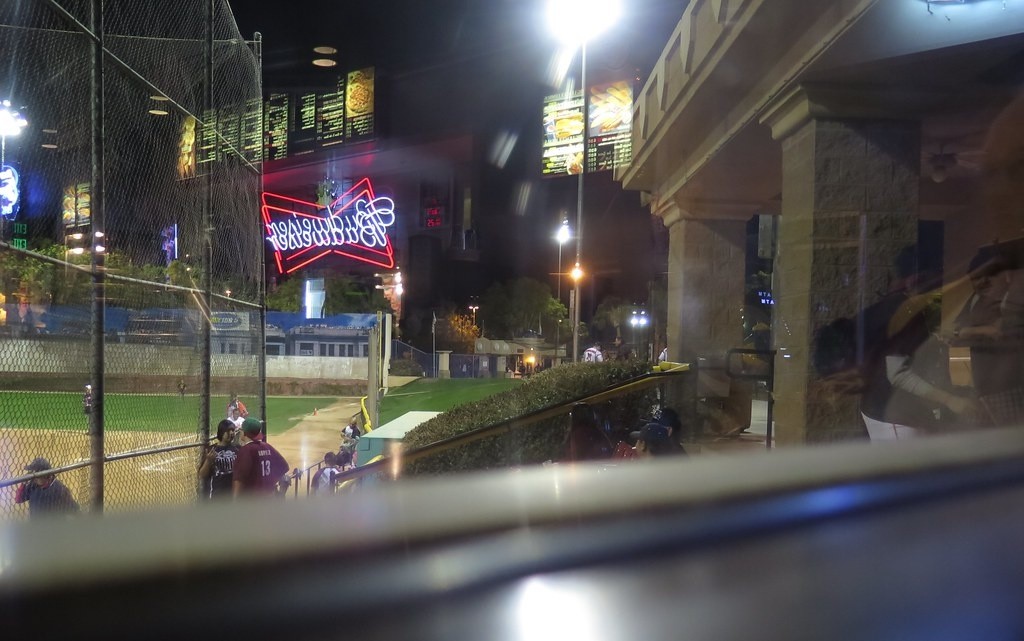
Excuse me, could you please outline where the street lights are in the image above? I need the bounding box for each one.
[549,0,609,365]
[554,220,570,366]
[468,306,479,325]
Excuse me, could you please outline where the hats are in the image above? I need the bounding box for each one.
[24,458,51,472]
[629,423,667,445]
[242,418,261,432]
[640,407,681,431]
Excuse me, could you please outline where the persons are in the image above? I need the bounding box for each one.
[658,340,667,361]
[82,385,92,435]
[16,459,81,521]
[463,360,473,377]
[543,402,612,464]
[614,337,632,362]
[814,242,1024,445]
[579,342,610,364]
[631,407,687,458]
[198,419,289,505]
[178,380,187,398]
[312,420,361,488]
[225,393,248,445]
